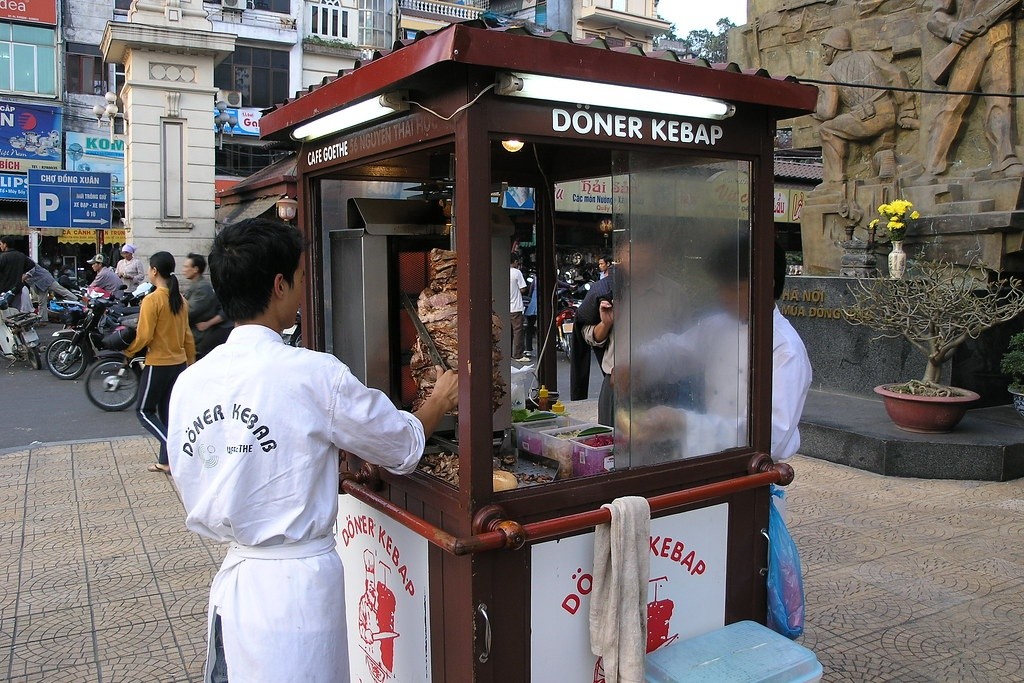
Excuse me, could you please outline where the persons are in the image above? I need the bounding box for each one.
[571,226,686,427]
[165,219,458,683]
[510,252,575,362]
[122,252,234,473]
[609,228,813,462]
[115,244,144,307]
[816,26,917,192]
[87,253,122,334]
[0,236,36,312]
[927,0,1024,176]
[54,264,72,323]
[22,257,89,326]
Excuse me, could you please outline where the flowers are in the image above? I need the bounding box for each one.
[869,199,919,241]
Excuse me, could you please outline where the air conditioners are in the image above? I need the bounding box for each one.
[216,89,242,108]
[221,0,246,12]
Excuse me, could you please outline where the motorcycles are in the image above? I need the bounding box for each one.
[282,308,304,347]
[0,276,148,411]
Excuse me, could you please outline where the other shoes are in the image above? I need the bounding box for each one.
[524,350,532,356]
[32,322,47,327]
[516,356,531,362]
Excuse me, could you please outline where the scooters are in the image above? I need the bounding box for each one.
[520,270,594,362]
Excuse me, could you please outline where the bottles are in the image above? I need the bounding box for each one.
[539,385,548,411]
[552,398,568,427]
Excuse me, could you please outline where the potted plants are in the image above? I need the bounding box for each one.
[1001,332,1024,420]
[838,253,1024,433]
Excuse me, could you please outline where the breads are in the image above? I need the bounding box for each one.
[493,471,518,490]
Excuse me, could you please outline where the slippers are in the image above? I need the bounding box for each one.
[147,463,170,472]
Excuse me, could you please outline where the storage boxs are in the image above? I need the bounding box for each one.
[511,411,624,480]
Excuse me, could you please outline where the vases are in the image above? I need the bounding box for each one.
[888,241,907,278]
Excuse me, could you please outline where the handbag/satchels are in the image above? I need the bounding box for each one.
[767,485,804,641]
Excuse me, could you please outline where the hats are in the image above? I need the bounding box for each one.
[86,254,106,264]
[122,243,137,253]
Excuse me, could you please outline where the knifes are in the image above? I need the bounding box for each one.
[398,286,450,376]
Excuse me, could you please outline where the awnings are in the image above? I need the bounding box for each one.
[215,197,280,226]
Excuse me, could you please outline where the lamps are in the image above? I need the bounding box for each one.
[93,92,129,148]
[215,101,237,150]
